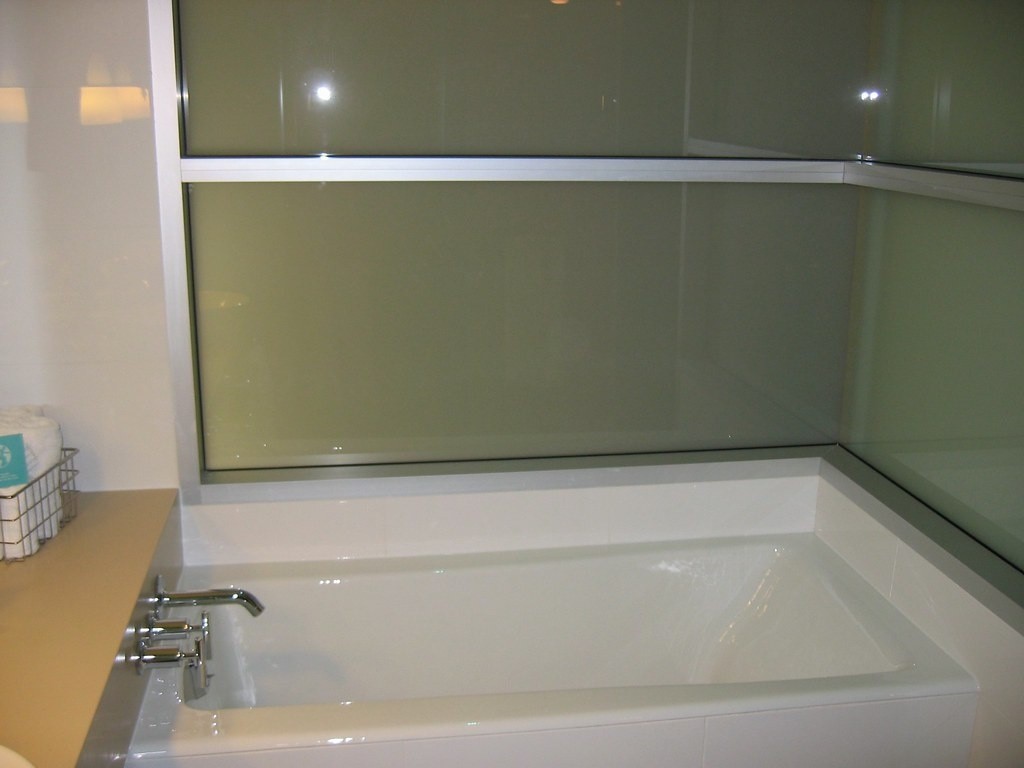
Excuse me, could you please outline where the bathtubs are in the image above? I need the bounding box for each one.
[124,529,983,768]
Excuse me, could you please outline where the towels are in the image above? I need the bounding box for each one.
[0,405,64,560]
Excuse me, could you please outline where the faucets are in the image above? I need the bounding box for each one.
[150,572,266,619]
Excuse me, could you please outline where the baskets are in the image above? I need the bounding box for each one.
[0,447,80,565]
